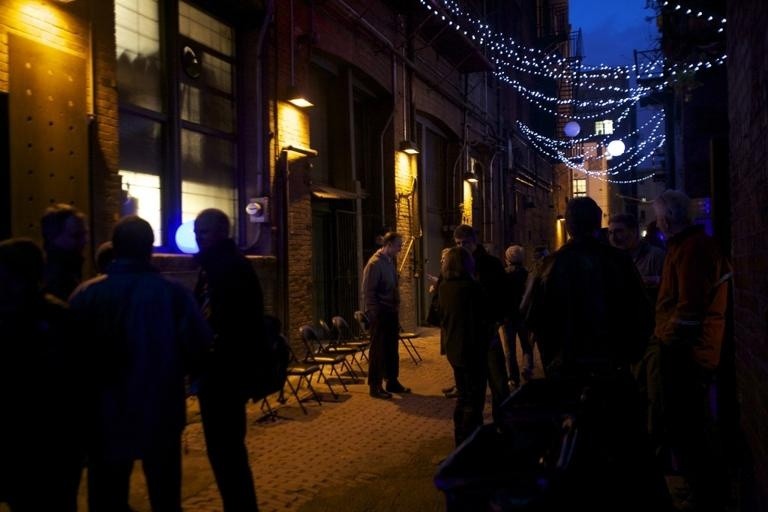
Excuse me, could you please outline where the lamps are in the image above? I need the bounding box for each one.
[464,171,479,182]
[286,93,316,109]
[400,140,420,155]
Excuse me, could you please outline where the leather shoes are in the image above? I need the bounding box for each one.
[370,387,392,398]
[386,383,410,393]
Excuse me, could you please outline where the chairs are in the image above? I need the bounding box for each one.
[260,310,422,422]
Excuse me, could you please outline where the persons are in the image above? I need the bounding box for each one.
[422,188,738,511]
[191,207,263,511]
[0,202,197,510]
[359,231,412,402]
